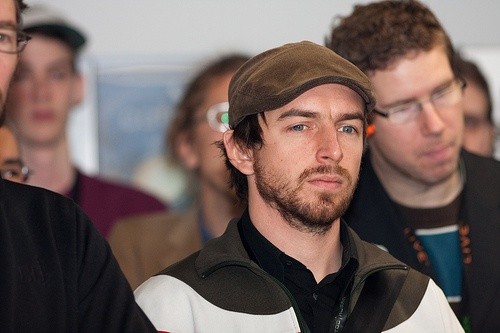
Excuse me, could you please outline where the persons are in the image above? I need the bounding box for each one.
[324,0,500,332]
[0,0,169,333]
[105,55,250,291]
[133,41,465,332]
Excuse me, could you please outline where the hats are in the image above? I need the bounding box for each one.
[228,41,377,126]
[19,8,88,49]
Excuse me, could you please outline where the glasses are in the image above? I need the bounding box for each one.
[184,102,230,133]
[369,71,467,125]
[4,165,32,183]
[0,24,33,55]
